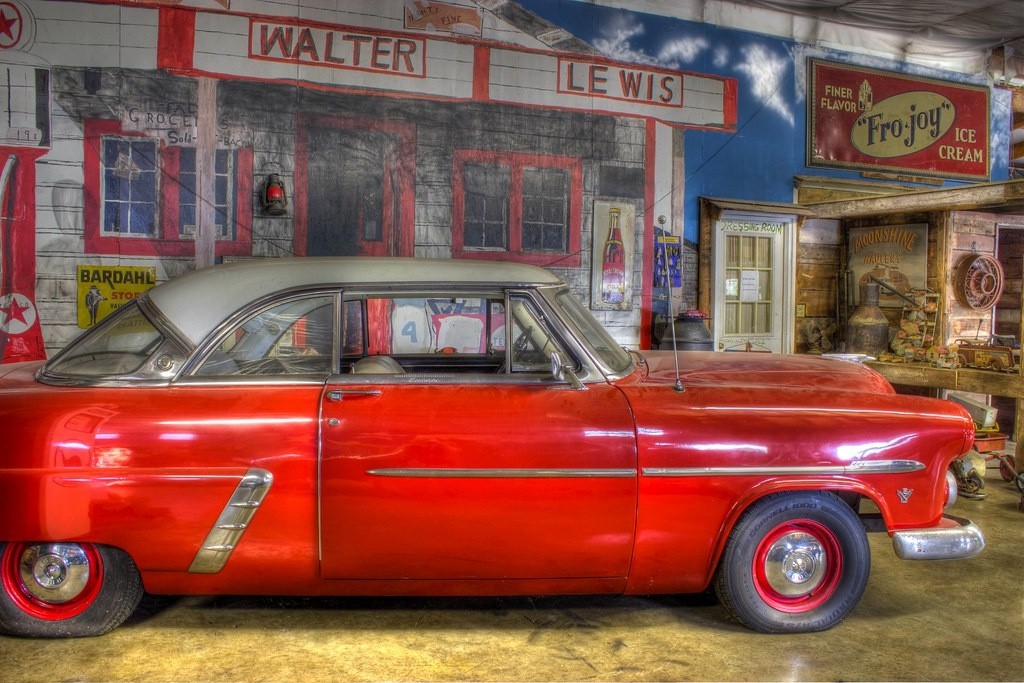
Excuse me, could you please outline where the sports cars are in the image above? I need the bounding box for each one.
[0,256,984,641]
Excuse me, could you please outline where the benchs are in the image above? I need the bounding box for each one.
[234,357,290,374]
[353,355,406,374]
